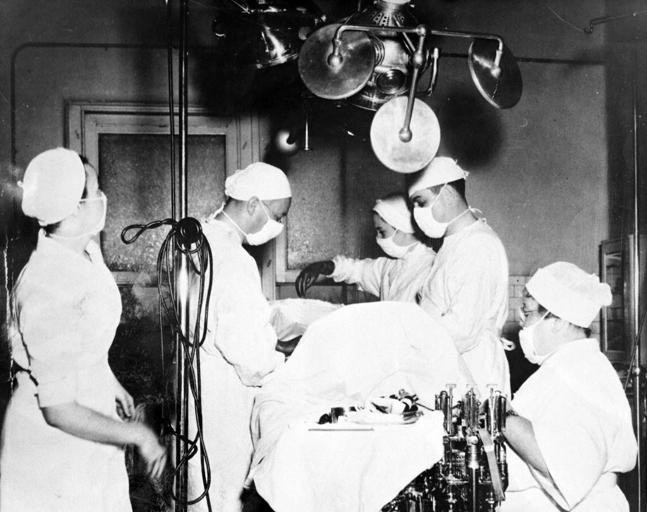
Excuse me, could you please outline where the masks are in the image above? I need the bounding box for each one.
[414,183,468,240]
[235,197,285,246]
[517,309,570,367]
[78,190,108,240]
[375,228,417,259]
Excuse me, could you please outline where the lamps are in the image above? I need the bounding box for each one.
[211,0,524,174]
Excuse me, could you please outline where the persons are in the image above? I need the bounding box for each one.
[162,158,292,511]
[292,194,432,304]
[397,155,520,404]
[3,145,169,512]
[487,262,639,511]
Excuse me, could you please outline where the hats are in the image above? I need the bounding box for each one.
[408,155,470,198]
[20,146,86,228]
[224,161,293,201]
[372,191,419,235]
[524,261,613,329]
